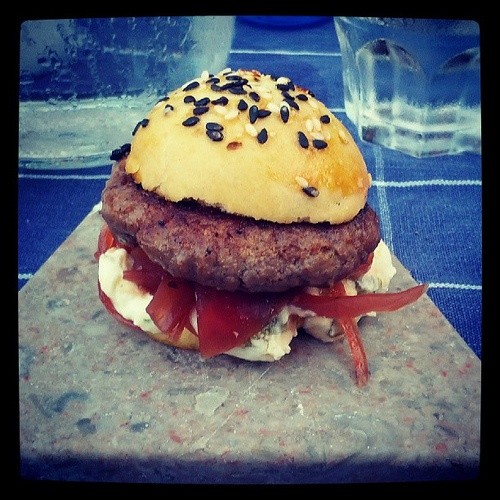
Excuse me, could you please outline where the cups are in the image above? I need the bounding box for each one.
[19,16,234,170]
[333,17,481,158]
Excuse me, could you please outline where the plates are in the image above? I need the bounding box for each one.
[19,199,482,478]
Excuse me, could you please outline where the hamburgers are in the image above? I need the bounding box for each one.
[94,67,429,384]
